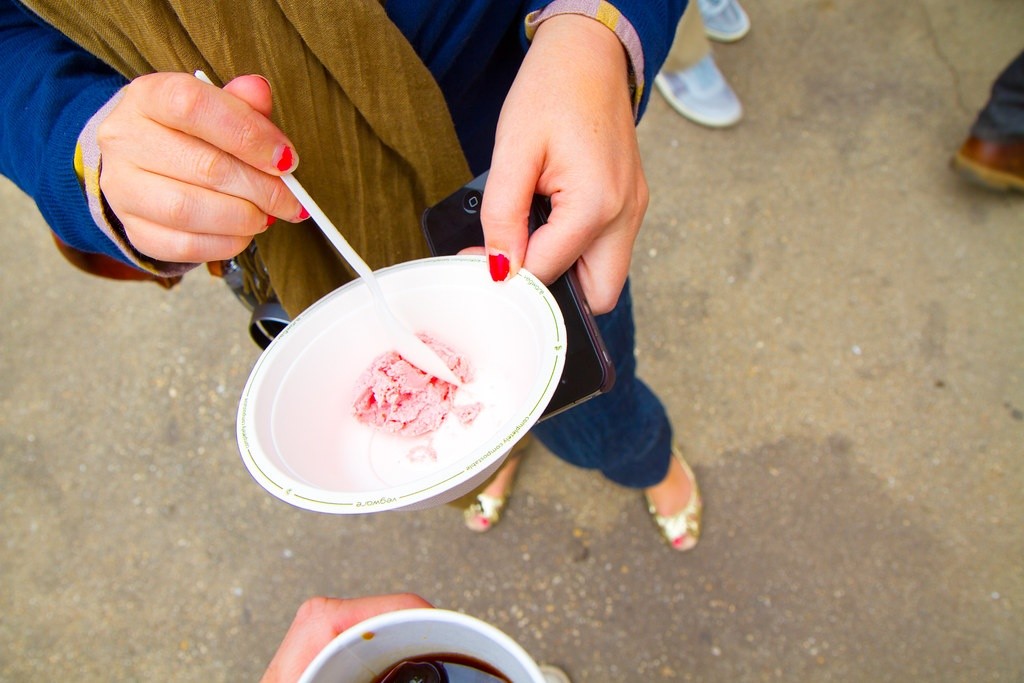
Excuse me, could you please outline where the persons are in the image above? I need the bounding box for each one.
[655,0,752,130]
[0,0,698,554]
[950,49,1023,196]
[256,592,437,683]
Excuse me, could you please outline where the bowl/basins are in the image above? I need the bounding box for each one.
[238,256,568,515]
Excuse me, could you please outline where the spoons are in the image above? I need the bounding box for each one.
[196,70,462,385]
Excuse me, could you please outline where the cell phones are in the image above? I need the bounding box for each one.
[422,167,616,427]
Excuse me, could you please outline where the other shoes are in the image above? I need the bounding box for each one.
[950,129,1024,188]
[463,447,527,532]
[698,0,752,44]
[653,53,741,127]
[644,447,704,553]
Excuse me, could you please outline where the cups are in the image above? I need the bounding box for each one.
[298,608,545,683]
